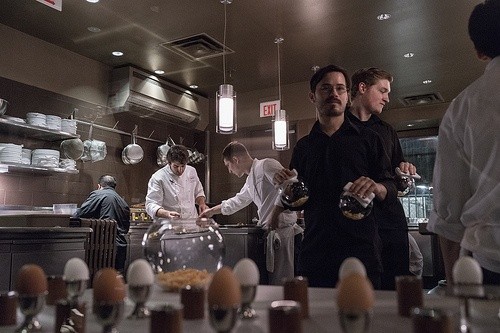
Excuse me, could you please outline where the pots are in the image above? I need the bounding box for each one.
[122,130,144,165]
[61,123,107,162]
[157,142,204,168]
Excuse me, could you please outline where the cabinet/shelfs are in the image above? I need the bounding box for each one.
[0,118,80,175]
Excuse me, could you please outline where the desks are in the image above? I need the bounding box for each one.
[0,223,500,333]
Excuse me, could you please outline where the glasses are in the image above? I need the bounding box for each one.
[311,84,350,93]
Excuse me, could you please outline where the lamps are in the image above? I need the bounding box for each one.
[272,37,290,151]
[216,0,238,135]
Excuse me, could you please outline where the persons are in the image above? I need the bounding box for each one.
[426,0,500,284]
[273,64,423,291]
[145,145,209,219]
[199,141,304,285]
[73,175,130,274]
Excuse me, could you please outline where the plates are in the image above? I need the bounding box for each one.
[6,112,78,134]
[0,143,60,168]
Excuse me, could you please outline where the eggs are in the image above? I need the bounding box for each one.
[338,257,366,282]
[16,264,48,294]
[208,265,242,306]
[233,259,260,285]
[127,260,153,285]
[452,257,482,284]
[65,258,87,281]
[335,272,375,312]
[92,267,126,303]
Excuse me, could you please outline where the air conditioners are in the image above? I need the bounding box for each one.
[110,67,210,131]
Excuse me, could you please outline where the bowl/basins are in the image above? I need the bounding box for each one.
[53,204,77,214]
[0,98,9,118]
